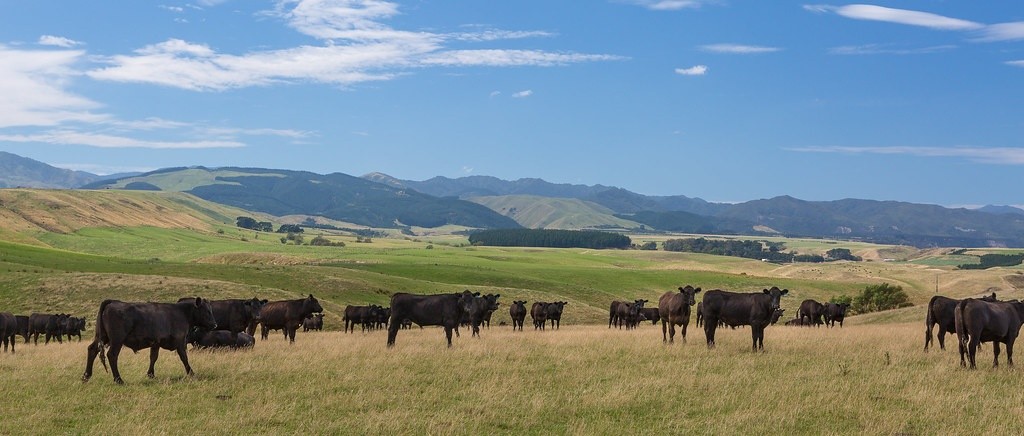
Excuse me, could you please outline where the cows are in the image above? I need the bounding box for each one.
[256,294,323,343]
[796,300,828,328]
[386,289,501,348]
[954,298,1024,370]
[923,292,996,352]
[342,303,413,334]
[658,285,702,343]
[785,316,825,326]
[0,312,16,352]
[822,302,850,328]
[703,286,789,354]
[176,296,268,350]
[303,313,325,332]
[509,300,527,331]
[609,299,661,330]
[546,300,568,330]
[14,313,86,346]
[530,301,548,331]
[81,296,218,385]
[696,302,785,329]
